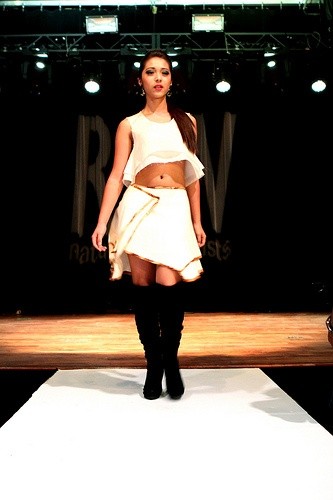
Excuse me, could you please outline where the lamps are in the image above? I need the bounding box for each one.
[34,51,328,95]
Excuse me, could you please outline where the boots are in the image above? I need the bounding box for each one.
[131,282,164,399]
[155,282,186,400]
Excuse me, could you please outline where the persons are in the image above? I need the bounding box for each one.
[91,49,207,399]
